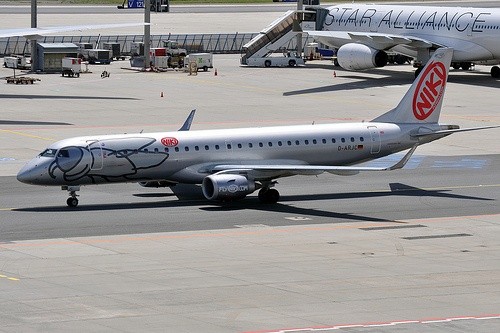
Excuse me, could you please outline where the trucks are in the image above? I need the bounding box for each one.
[167,49,214,71]
[246,51,304,67]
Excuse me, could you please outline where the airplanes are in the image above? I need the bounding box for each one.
[16,46,500,205]
[302,5,500,79]
